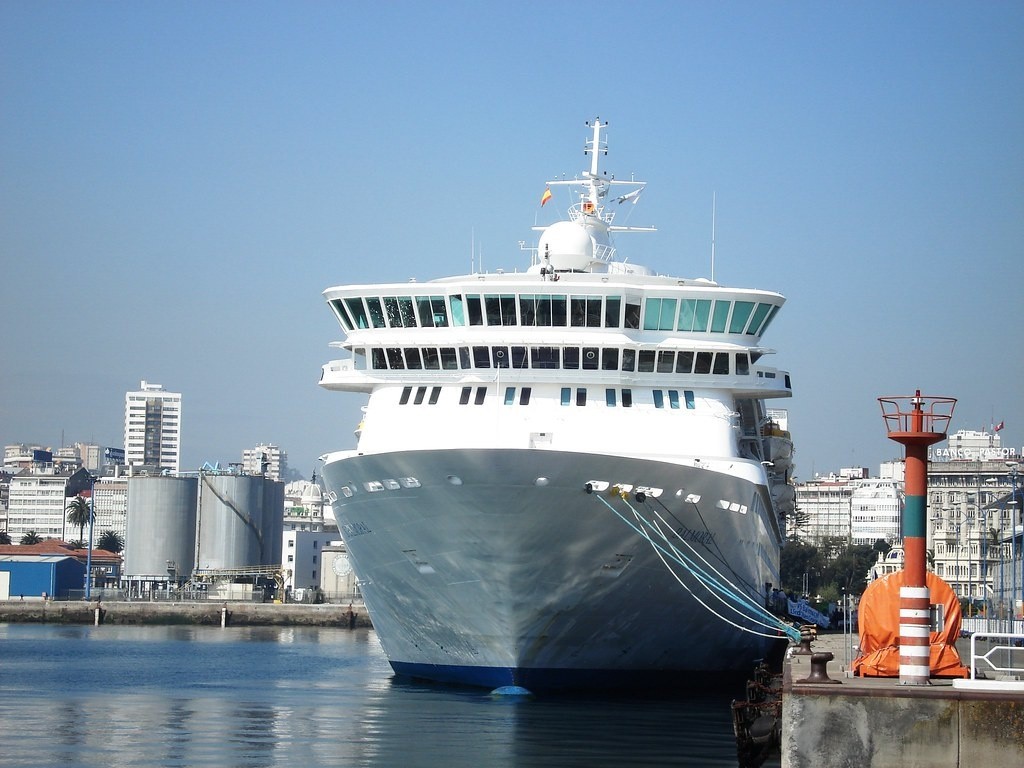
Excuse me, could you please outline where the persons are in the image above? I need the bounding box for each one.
[772,588,858,632]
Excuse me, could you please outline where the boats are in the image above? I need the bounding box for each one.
[314,114,795,696]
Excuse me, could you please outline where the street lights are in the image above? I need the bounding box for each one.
[986,478,1024,622]
[965,490,1024,619]
[952,501,1018,618]
[929,516,985,599]
[942,507,999,618]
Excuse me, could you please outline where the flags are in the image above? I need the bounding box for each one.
[994,421,1005,431]
[541,187,552,206]
